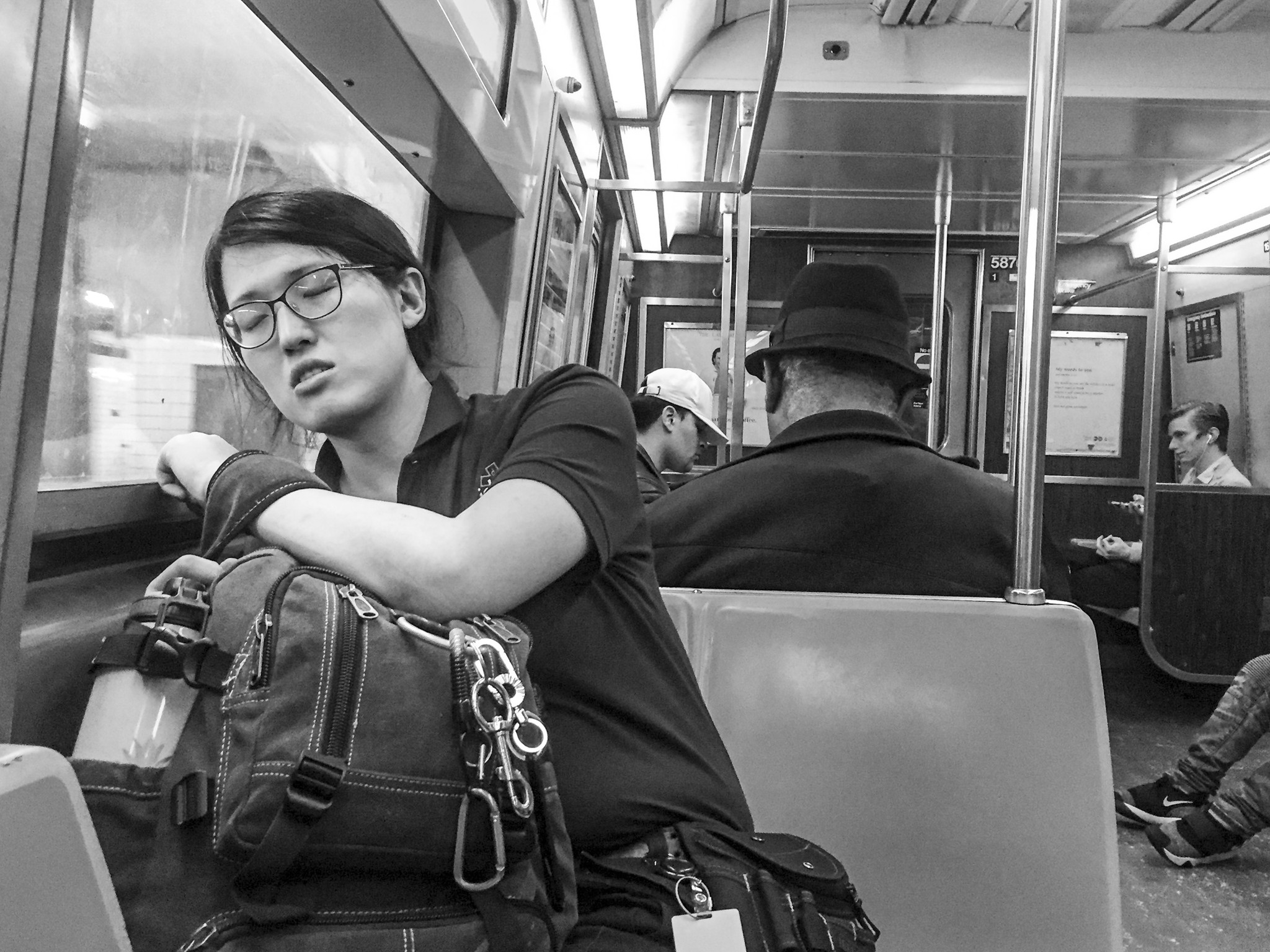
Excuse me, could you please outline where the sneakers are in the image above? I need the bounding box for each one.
[1114,774,1208,829]
[1145,812,1241,866]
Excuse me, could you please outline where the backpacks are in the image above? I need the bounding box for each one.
[59,449,581,952]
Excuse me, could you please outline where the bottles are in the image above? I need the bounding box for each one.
[68,566,215,774]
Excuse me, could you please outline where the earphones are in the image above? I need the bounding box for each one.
[1206,435,1212,445]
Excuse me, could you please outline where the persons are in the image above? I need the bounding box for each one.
[1113,653,1270,868]
[1061,399,1252,608]
[706,348,732,401]
[644,263,1073,602]
[153,188,756,951]
[630,368,712,503]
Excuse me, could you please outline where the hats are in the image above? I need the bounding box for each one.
[640,368,730,447]
[745,263,932,393]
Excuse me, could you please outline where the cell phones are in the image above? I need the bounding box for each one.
[1111,500,1143,508]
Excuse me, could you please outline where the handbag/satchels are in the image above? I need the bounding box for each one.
[690,830,881,952]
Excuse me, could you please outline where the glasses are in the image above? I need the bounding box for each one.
[215,262,373,349]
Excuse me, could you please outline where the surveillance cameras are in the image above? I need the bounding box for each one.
[568,77,581,92]
[629,274,635,282]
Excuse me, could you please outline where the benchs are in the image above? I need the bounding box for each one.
[73,587,1124,952]
